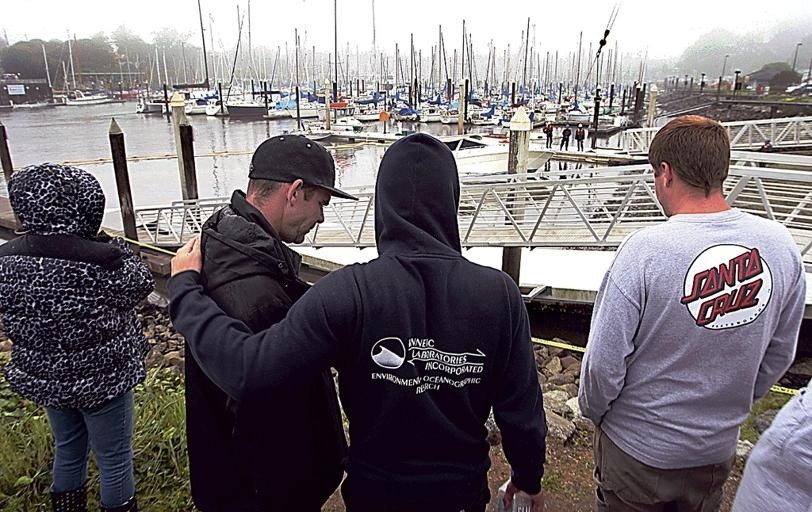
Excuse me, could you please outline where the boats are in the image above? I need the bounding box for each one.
[427,133,558,178]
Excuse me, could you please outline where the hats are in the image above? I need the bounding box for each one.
[245,133,360,205]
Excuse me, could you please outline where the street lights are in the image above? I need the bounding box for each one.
[662,72,707,91]
[591,85,602,147]
[792,41,804,71]
[721,55,732,80]
[734,69,743,96]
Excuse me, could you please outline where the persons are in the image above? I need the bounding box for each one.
[1,163,155,511]
[559,124,571,151]
[574,124,585,152]
[167,133,549,511]
[185,133,347,512]
[545,123,553,148]
[575,115,806,512]
